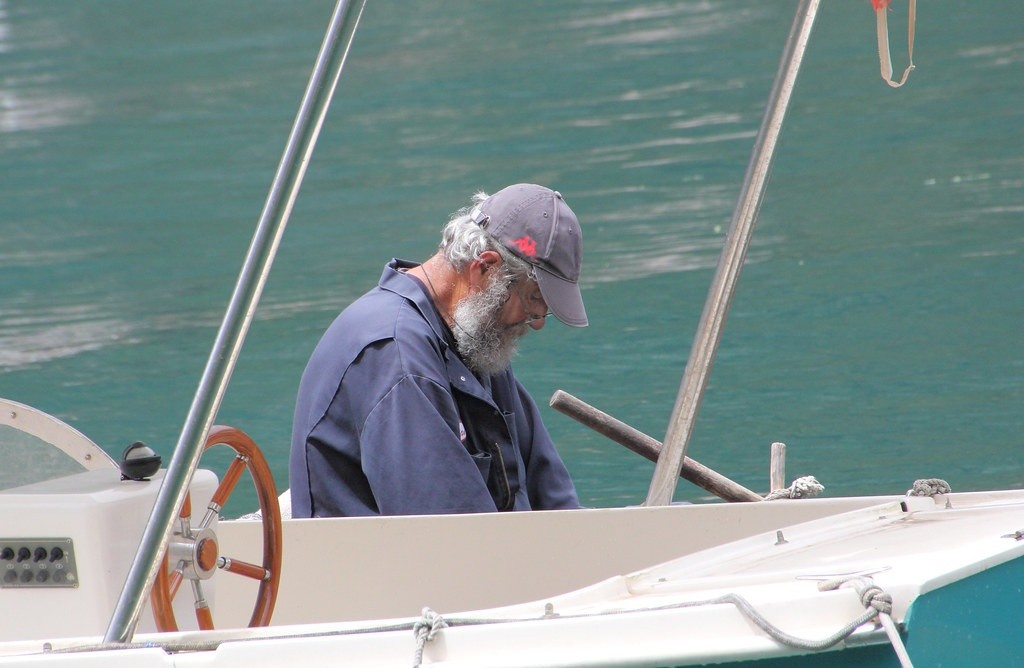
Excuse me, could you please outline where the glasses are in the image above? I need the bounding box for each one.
[510,279,553,326]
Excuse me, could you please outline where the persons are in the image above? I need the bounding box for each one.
[285,181,591,520]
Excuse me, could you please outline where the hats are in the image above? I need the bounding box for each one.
[469,183,589,328]
